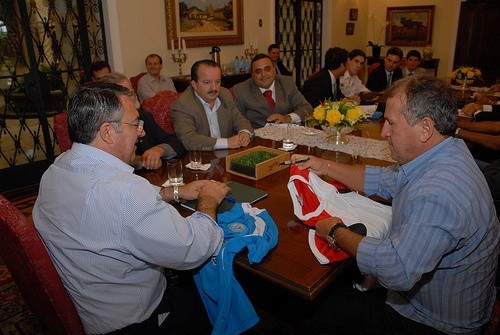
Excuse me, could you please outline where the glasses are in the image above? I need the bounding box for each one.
[98,119,144,133]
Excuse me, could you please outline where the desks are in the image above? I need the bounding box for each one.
[366,56,440,77]
[142,88,497,302]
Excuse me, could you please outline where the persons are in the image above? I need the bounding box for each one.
[170,59,255,151]
[268,44,290,75]
[453,84,500,200]
[137,54,176,102]
[301,47,360,108]
[366,46,404,92]
[291,75,496,335]
[90,61,112,82]
[233,53,314,129]
[101,73,187,175]
[340,49,370,97]
[32,81,231,335]
[403,50,426,76]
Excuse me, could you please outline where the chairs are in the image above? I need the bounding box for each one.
[0,194,87,335]
[54,112,73,152]
[142,90,183,135]
[129,73,146,104]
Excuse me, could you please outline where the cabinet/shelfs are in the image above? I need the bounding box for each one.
[171,74,251,93]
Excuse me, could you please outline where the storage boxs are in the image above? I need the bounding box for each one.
[224,145,290,181]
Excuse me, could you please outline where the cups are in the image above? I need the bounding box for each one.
[305,116,314,136]
[189,144,202,169]
[167,158,183,185]
[283,127,295,149]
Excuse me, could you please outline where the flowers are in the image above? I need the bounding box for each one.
[364,8,389,48]
[304,99,371,133]
[450,64,482,82]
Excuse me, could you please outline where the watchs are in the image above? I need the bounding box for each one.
[327,223,347,251]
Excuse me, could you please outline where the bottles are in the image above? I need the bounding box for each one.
[232,56,252,74]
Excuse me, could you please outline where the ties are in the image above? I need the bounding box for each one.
[262,90,275,112]
[333,83,337,99]
[387,72,392,86]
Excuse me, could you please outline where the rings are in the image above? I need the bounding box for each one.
[211,180,215,182]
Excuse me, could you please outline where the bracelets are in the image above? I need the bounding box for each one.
[174,186,178,201]
[455,128,461,138]
[326,160,331,176]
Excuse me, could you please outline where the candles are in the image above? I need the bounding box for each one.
[171,38,175,52]
[182,38,187,53]
[245,34,258,49]
[178,35,181,50]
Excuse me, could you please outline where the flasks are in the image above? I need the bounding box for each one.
[209,46,221,67]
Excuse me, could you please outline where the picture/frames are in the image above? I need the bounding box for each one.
[163,0,245,50]
[345,22,354,35]
[384,4,436,47]
[349,9,358,20]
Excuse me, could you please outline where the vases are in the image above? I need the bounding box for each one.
[372,46,381,56]
[455,79,474,88]
[320,125,355,146]
[422,43,433,60]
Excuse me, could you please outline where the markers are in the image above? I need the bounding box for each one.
[224,196,236,202]
[282,157,309,164]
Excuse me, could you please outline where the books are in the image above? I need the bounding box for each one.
[181,182,268,211]
